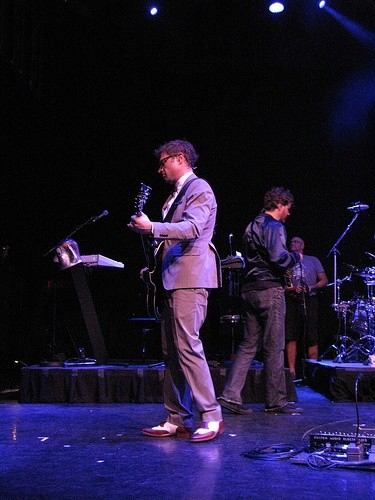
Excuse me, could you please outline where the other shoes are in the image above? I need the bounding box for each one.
[219,399,252,414]
[274,404,303,413]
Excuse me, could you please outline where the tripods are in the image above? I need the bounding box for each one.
[317,265,375,365]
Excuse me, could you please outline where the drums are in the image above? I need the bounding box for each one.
[350,298,375,335]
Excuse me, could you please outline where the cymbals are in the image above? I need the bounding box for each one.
[353,272,375,279]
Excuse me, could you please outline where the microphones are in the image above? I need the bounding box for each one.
[347,205,369,211]
[365,251,375,258]
[93,210,108,222]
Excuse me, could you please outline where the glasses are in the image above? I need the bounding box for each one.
[289,241,301,244]
[159,153,182,167]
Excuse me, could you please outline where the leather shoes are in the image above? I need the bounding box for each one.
[142,422,186,436]
[190,420,225,442]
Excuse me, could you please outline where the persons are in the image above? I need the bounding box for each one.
[219,187,303,415]
[127,139,225,442]
[284,237,329,379]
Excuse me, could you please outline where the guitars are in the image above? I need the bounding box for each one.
[134,181,165,319]
